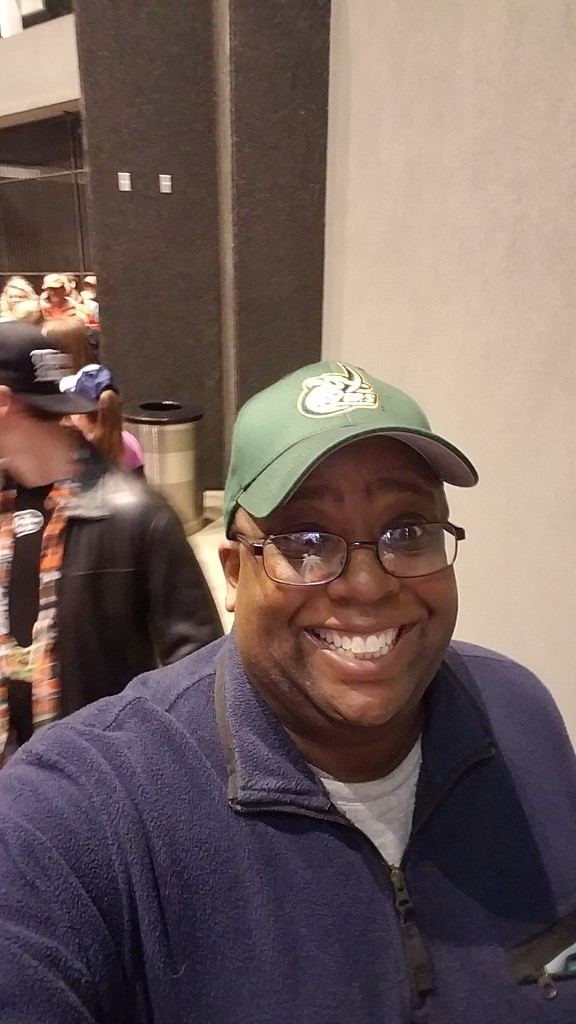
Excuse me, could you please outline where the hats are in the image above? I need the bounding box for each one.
[1,323,103,415]
[224,360,479,536]
[40,273,64,290]
[58,364,112,403]
[84,276,97,285]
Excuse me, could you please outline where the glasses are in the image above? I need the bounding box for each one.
[230,519,466,586]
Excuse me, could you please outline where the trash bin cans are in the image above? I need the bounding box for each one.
[119,399,206,537]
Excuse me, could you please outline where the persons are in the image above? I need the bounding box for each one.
[0,272,147,484]
[0,321,224,769]
[0,360,576,1024]
